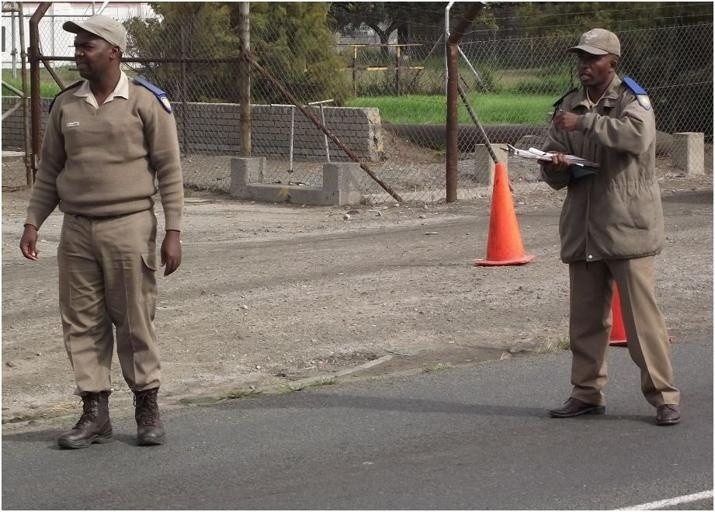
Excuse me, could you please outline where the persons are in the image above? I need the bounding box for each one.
[538,28,682,426]
[20,15,184,450]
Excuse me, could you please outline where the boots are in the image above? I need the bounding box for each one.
[58,389,113,450]
[133,388,166,448]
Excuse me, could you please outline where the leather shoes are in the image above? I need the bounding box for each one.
[550,398,609,420]
[656,404,682,427]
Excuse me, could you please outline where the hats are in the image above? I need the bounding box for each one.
[565,28,621,58]
[62,15,128,52]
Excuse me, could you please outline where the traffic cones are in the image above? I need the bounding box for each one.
[607,281,628,347]
[470,157,538,268]
